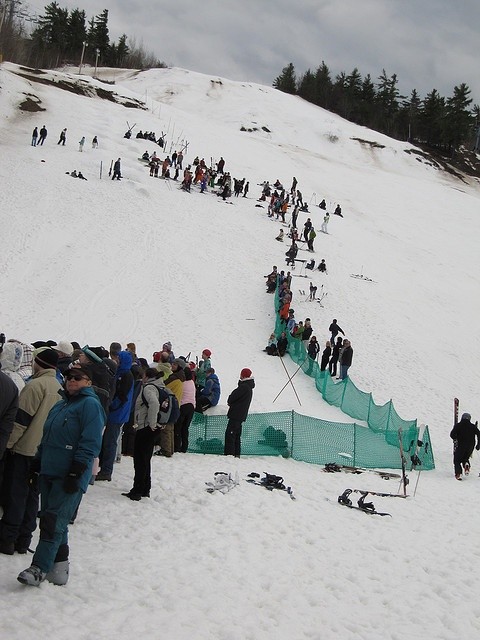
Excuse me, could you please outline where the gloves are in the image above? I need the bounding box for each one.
[63,462,88,493]
[25,459,41,494]
[476,444,480,450]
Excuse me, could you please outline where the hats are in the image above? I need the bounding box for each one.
[204,368,214,373]
[203,349,211,357]
[241,369,251,376]
[173,358,185,369]
[62,363,93,381]
[163,342,171,349]
[34,349,59,370]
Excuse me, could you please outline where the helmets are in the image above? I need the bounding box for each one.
[462,414,470,420]
[153,351,160,361]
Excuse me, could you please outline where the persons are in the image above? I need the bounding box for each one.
[78,137,85,152]
[321,212,330,233]
[275,229,284,242]
[321,341,331,378]
[341,341,353,381]
[263,266,320,376]
[259,180,289,222]
[336,339,348,379]
[318,259,326,272]
[58,128,67,146]
[334,204,343,217]
[111,158,121,180]
[144,131,149,139]
[71,170,77,178]
[329,337,342,376]
[450,413,480,481]
[176,151,183,170]
[165,169,171,178]
[150,133,157,143]
[0,329,220,587]
[291,189,296,205]
[292,205,299,228]
[318,199,326,210]
[162,159,169,176]
[142,151,150,163]
[329,319,345,346]
[124,130,131,139]
[150,151,163,162]
[77,172,87,180]
[31,127,38,146]
[296,190,303,208]
[184,156,249,200]
[299,202,308,212]
[171,151,177,169]
[136,131,144,139]
[166,156,172,167]
[93,136,98,146]
[158,137,163,147]
[148,132,152,140]
[303,218,312,241]
[285,239,298,266]
[175,162,180,181]
[224,368,255,459]
[306,227,316,252]
[290,177,298,194]
[37,125,47,145]
[149,162,159,176]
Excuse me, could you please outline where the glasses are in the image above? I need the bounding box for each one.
[66,374,90,381]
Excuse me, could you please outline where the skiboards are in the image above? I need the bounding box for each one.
[354,426,409,498]
[205,472,241,495]
[100,160,113,179]
[337,464,402,479]
[243,471,296,501]
[285,258,307,263]
[163,141,174,154]
[453,398,459,473]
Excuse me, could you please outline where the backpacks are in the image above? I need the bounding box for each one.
[141,383,180,425]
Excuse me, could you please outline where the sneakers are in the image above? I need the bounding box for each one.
[456,474,462,481]
[95,470,111,482]
[17,565,47,587]
[122,492,141,501]
[46,561,69,586]
[143,488,150,496]
[464,462,469,475]
[16,544,27,553]
[0,543,14,554]
